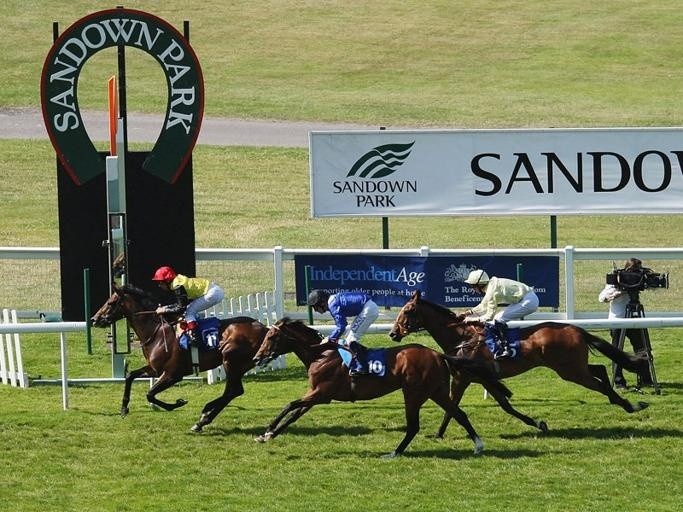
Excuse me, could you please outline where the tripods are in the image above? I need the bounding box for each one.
[611,296,661,394]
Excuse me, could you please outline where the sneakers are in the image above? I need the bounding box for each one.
[613,378,662,391]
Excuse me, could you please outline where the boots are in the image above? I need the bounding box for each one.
[494,322,513,360]
[347,340,368,377]
[186,328,203,347]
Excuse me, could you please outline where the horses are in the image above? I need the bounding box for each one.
[88,281,272,434]
[110,253,127,279]
[387,290,655,440]
[252,316,485,459]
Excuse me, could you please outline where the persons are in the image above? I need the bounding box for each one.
[306,289,379,375]
[596,258,661,389]
[455,269,539,358]
[151,266,224,345]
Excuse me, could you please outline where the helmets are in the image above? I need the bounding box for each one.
[463,268,490,285]
[151,266,177,282]
[305,289,329,307]
